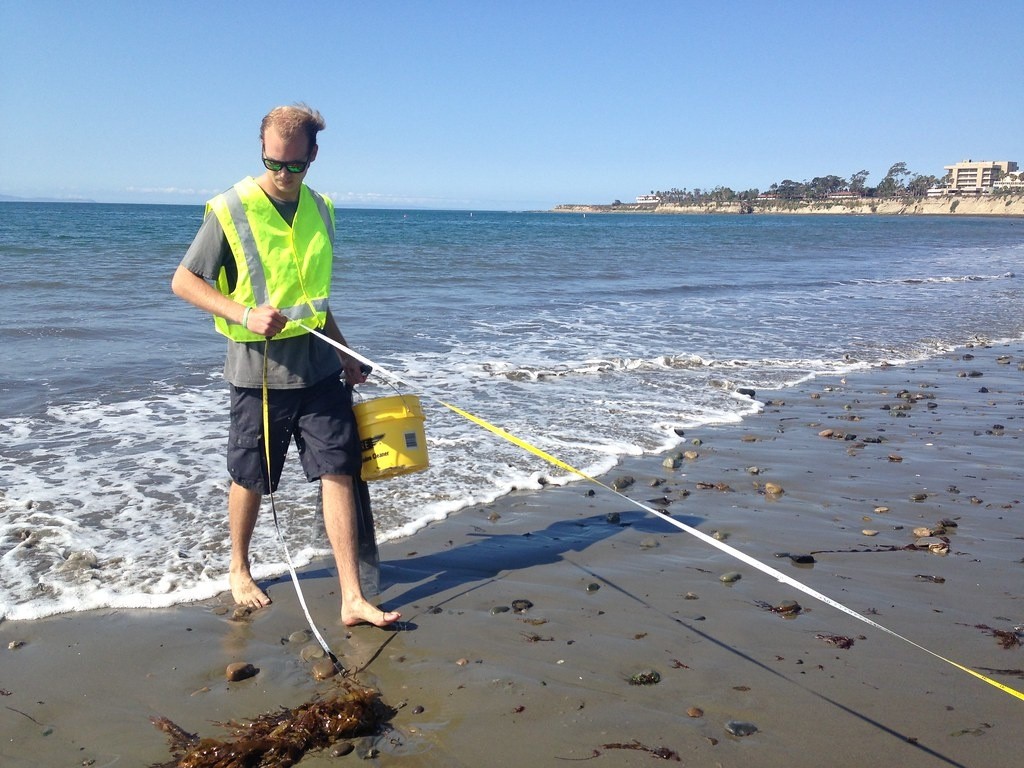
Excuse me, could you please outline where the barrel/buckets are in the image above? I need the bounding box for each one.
[339,371,430,481]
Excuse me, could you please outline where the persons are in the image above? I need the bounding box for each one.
[173,106,403,627]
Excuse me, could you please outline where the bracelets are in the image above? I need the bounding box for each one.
[241,306,252,329]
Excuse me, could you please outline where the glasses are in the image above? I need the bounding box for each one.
[261,143,313,173]
